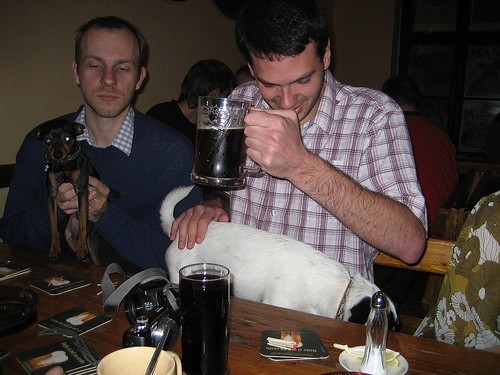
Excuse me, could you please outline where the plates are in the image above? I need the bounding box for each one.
[338,346,409,375]
[97,347,182,375]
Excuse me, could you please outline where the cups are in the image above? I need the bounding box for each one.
[1,262,38,356]
[189,96,264,188]
[180,266,229,375]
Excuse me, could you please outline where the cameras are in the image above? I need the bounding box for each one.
[123,305,179,351]
[123,276,181,325]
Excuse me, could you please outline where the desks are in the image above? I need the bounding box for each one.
[0,242,500,375]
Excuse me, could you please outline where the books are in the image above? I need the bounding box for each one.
[0,335,101,375]
[0,260,32,282]
[35,305,113,339]
[260,330,330,362]
[407,0,500,167]
[29,272,92,295]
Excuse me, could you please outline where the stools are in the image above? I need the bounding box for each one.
[372,234,455,338]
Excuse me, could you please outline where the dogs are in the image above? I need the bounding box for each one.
[34,118,107,267]
[158,183,404,335]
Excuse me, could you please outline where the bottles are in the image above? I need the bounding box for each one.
[358,291,389,373]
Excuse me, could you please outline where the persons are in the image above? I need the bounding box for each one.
[0,13,206,278]
[380,75,460,242]
[433,111,500,238]
[234,64,257,90]
[169,0,429,286]
[142,58,236,148]
[410,189,500,356]
